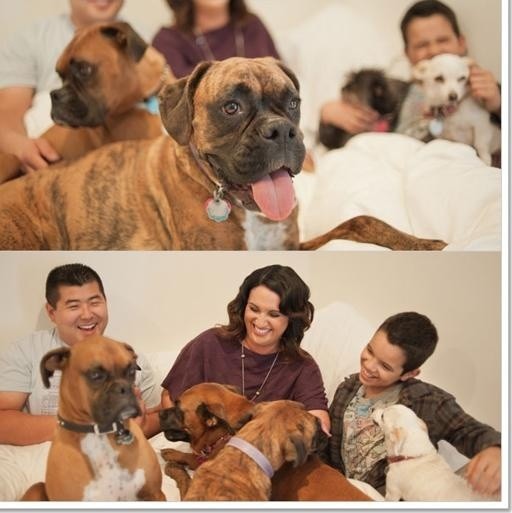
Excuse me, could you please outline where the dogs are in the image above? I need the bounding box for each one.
[180,398,329,500]
[370,403,484,500]
[318,67,409,149]
[20,334,166,500]
[410,53,500,165]
[157,382,374,501]
[0,55,448,250]
[0,20,178,184]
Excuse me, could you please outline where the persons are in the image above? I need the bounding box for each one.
[326,312,501,501]
[319,0,501,167]
[0,263,162,447]
[160,265,332,439]
[151,0,281,79]
[0,0,146,176]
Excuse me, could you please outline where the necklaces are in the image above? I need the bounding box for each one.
[194,27,245,60]
[241,342,281,401]
